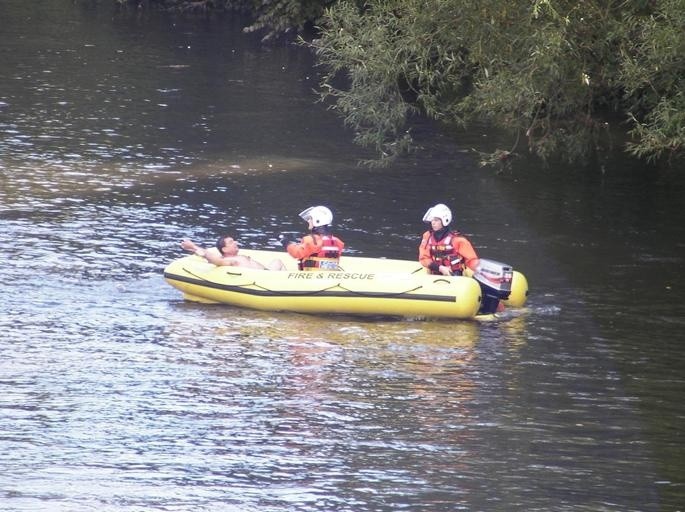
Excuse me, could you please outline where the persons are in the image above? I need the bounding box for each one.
[180,235,286,271]
[418,203,480,277]
[286,206,345,270]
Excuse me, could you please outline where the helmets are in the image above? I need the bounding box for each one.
[307,205,334,227]
[429,203,453,227]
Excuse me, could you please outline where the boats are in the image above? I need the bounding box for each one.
[163,246,529,321]
